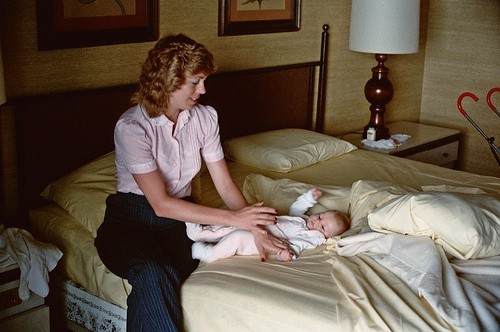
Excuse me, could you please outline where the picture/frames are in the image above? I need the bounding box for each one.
[36,0,159,51]
[218,0,301,36]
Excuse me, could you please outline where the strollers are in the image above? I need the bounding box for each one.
[456,87,500,166]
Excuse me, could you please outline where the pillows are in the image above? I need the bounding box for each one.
[40,150,116,238]
[223,128,359,173]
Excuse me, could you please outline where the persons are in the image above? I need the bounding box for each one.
[94,33,295,332]
[185,185,350,262]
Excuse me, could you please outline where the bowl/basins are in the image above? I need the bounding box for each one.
[391,134,411,142]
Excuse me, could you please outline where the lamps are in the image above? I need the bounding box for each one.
[349,0,420,140]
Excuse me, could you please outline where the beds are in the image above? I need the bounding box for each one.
[13,25,500,332]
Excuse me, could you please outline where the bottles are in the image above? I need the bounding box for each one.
[367,128,376,142]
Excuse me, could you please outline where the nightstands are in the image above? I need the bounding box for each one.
[0,255,65,332]
[343,119,461,170]
